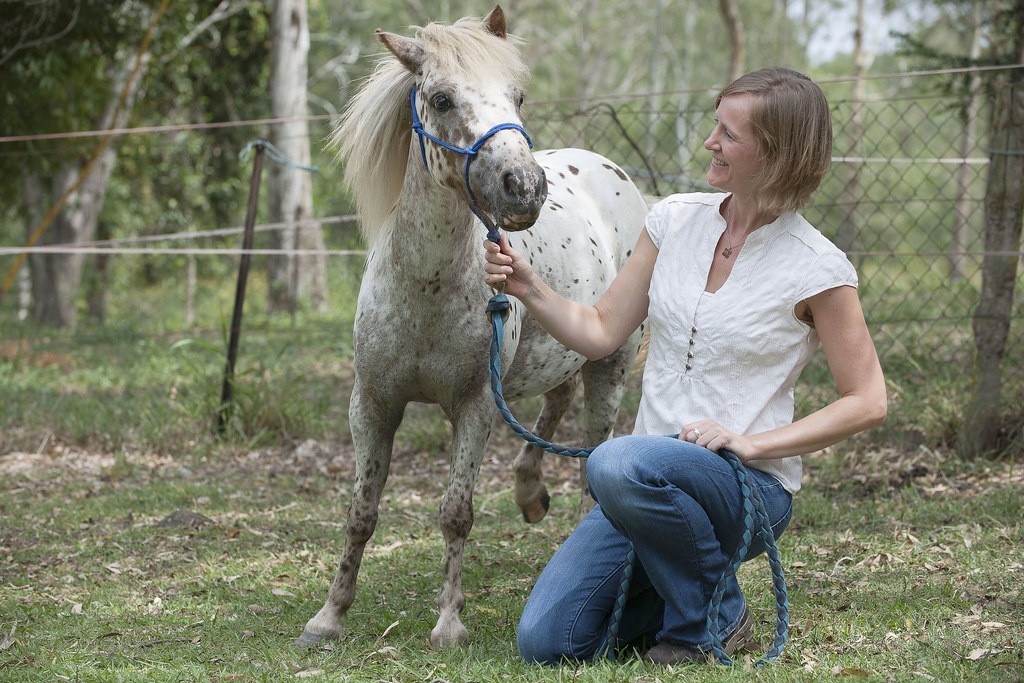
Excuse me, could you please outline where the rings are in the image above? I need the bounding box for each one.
[693,429,703,440]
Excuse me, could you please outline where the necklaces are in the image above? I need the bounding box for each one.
[722,227,745,259]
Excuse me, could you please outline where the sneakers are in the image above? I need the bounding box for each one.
[646,603,754,664]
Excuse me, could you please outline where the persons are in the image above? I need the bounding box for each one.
[480,66,888,667]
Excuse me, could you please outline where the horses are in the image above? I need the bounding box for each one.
[286,5,664,649]
